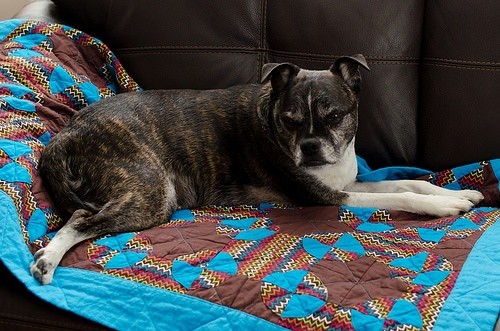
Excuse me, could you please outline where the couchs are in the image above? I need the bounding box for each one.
[0,0,500,331]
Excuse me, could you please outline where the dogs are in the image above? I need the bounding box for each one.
[28,53,484,286]
